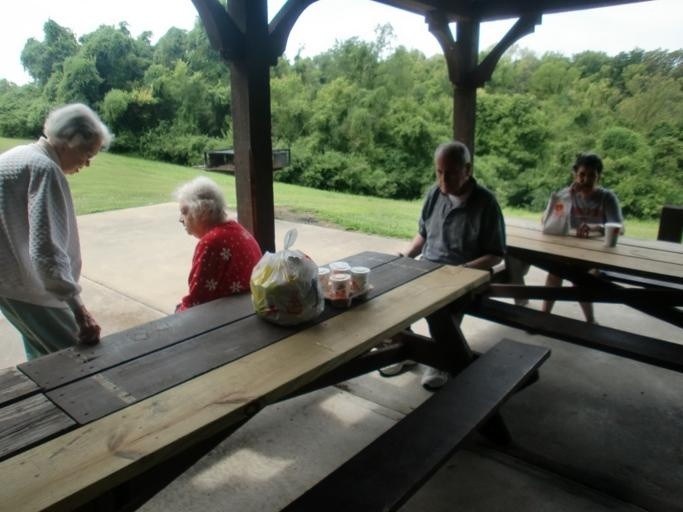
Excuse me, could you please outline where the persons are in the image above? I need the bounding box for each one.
[0,102,114,361]
[538,153,626,325]
[174,175,262,313]
[378,140,508,390]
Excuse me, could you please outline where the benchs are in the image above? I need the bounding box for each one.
[460,296,682,372]
[287,332,554,510]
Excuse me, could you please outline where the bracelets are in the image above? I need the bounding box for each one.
[462,262,468,267]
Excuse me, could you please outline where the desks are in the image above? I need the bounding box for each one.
[491,217,683,328]
[2,247,491,512]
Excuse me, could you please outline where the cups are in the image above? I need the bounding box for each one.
[315,261,370,298]
[604,223,622,246]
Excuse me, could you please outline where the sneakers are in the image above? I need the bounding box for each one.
[379,359,417,378]
[421,366,448,391]
